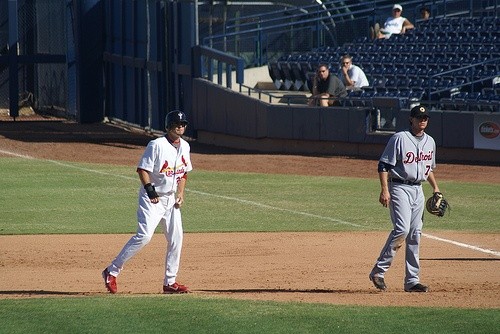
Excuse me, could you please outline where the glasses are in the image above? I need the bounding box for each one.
[173,122,187,127]
[416,116,429,122]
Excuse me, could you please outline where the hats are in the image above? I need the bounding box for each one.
[410,106,430,121]
[393,4,402,11]
[165,112,189,128]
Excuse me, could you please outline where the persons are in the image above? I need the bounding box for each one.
[338,55,369,90]
[370,4,414,40]
[102,111,192,294]
[421,9,430,19]
[308,65,346,107]
[369,105,441,292]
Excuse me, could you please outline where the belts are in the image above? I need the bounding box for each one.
[390,177,419,185]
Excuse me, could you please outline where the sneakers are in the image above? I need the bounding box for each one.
[163,282,189,293]
[369,274,386,290]
[102,268,117,294]
[405,283,428,292]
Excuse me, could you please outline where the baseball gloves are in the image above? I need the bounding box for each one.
[426,192,448,218]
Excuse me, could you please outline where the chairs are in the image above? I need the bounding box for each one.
[267,16,500,113]
